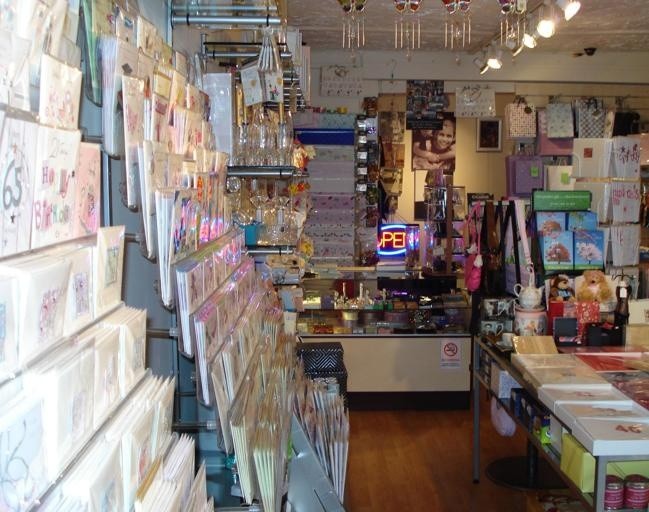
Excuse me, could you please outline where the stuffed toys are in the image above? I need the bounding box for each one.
[549,275,575,303]
[576,269,611,303]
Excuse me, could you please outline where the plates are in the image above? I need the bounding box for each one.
[496,341,510,350]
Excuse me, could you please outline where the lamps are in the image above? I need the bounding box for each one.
[469,1,581,75]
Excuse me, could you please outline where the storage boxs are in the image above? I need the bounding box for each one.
[478,349,595,494]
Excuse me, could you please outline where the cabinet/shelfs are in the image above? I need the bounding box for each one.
[531,188,627,346]
[80,0,349,512]
[425,175,467,278]
[294,303,472,411]
[470,335,649,512]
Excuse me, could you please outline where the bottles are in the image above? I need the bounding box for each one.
[514,305,548,339]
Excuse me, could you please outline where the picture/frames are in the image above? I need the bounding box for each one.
[475,118,502,153]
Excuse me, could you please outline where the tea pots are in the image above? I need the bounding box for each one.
[514,284,546,308]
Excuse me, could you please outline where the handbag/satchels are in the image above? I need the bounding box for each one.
[504,95,640,199]
[464,252,535,296]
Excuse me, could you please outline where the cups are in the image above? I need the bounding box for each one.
[237,121,295,167]
[502,333,515,346]
[481,321,504,336]
[484,299,504,316]
[240,225,257,247]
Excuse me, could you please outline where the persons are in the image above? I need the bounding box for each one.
[413,128,455,162]
[412,119,456,170]
